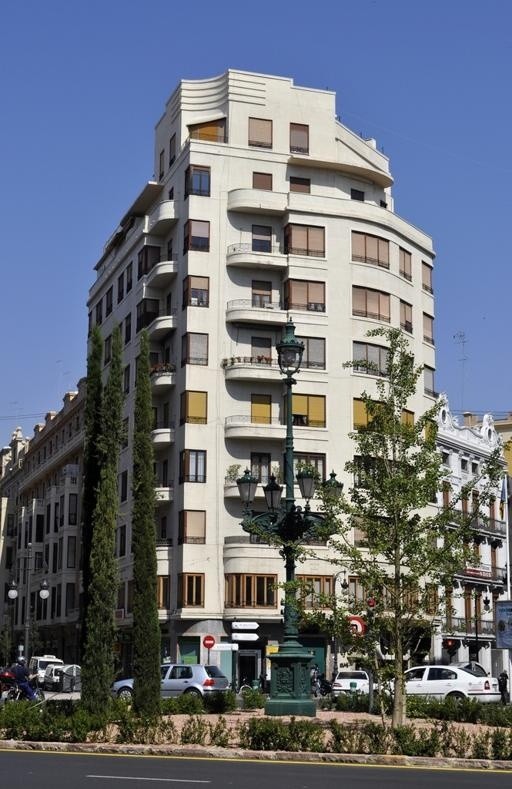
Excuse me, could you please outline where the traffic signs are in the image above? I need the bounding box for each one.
[231,633,259,641]
[232,621,259,631]
[210,642,239,651]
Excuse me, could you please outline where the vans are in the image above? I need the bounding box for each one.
[44,665,68,692]
[29,655,64,683]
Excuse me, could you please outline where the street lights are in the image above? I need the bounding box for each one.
[331,570,349,674]
[230,316,344,640]
[8,541,50,670]
[476,586,490,661]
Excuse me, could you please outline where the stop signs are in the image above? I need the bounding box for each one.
[203,635,215,649]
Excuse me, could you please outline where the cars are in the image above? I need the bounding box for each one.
[331,670,369,699]
[112,664,228,712]
[443,662,490,680]
[373,665,502,707]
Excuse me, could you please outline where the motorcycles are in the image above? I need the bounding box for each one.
[499,676,507,691]
[0,671,44,705]
[316,673,332,697]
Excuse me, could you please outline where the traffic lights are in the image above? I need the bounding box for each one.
[442,639,460,649]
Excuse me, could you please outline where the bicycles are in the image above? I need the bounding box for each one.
[240,674,267,698]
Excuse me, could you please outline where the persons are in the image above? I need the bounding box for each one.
[498,669,509,704]
[9,655,42,704]
[309,663,322,689]
